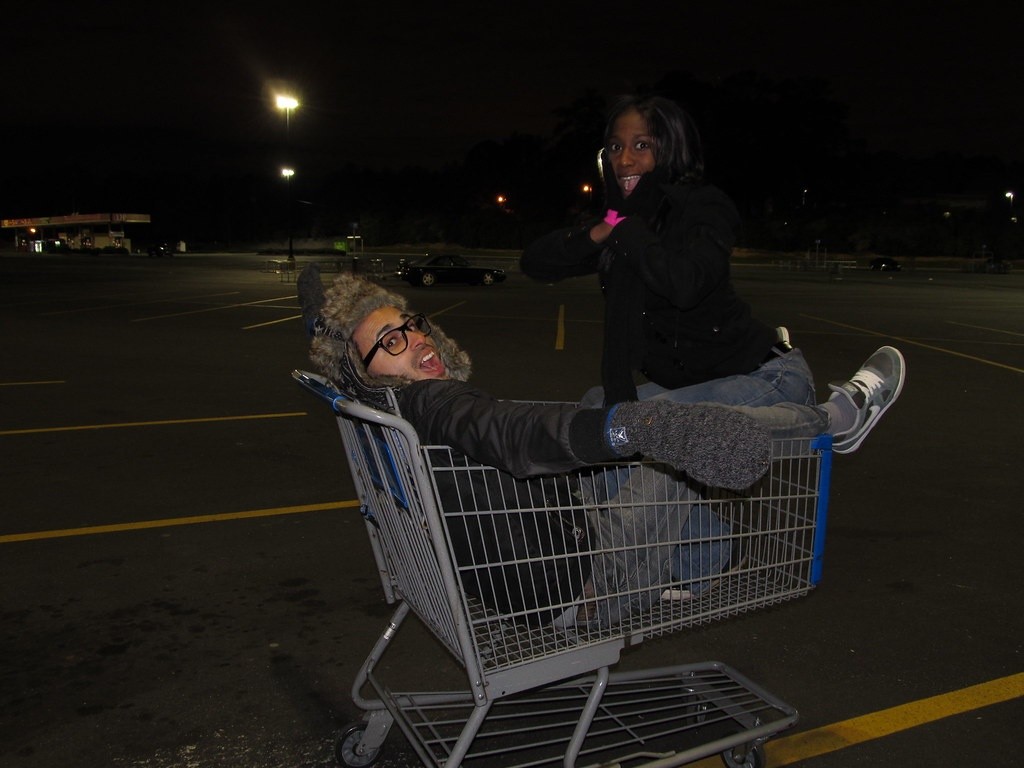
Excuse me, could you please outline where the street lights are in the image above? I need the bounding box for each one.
[276,95,299,260]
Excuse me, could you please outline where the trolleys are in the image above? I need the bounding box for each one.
[291,363,833,768]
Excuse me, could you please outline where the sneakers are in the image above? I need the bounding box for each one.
[830,346,905,454]
[661,542,750,603]
[758,327,790,371]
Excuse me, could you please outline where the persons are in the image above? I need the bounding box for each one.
[526,92,796,404]
[304,269,908,630]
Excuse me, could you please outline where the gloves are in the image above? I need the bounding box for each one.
[602,398,770,490]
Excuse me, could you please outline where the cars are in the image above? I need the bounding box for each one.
[866,258,905,271]
[393,253,505,287]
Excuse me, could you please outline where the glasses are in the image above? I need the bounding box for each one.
[363,312,432,372]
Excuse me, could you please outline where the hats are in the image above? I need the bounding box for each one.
[308,271,473,405]
